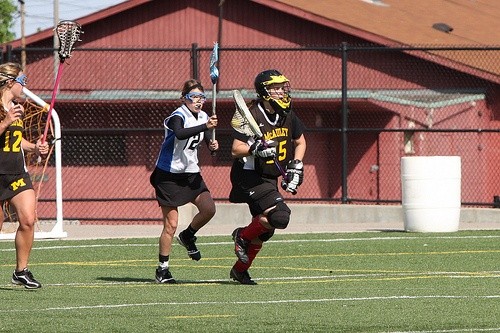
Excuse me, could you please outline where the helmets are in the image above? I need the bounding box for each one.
[254,69,291,111]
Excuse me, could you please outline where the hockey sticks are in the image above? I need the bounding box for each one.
[209,41,219,156]
[232,88,298,196]
[38,20,81,163]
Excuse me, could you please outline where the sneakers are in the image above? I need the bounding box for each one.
[12,267,40,288]
[232,228,249,263]
[177,231,201,260]
[155,268,175,283]
[230,267,256,285]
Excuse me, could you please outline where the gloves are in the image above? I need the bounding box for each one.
[249,140,278,159]
[281,160,304,193]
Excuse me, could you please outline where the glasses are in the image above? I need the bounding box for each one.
[17,74,27,85]
[187,92,206,103]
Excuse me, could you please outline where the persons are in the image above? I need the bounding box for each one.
[230,69,306,286]
[0,63,49,289]
[151,79,219,284]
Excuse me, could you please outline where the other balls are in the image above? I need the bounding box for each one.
[231,119,241,128]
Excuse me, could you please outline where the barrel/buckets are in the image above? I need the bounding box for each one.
[401,156,462,232]
